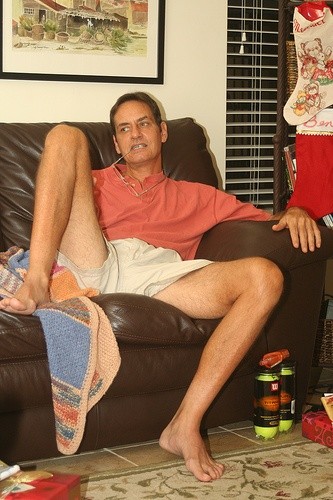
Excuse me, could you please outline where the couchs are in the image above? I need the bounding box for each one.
[0,118,333,463]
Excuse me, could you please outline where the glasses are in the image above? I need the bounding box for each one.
[108,147,170,198]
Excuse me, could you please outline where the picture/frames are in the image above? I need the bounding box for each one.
[0,0,165,84]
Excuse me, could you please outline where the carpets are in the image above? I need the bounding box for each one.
[80,437,333,500]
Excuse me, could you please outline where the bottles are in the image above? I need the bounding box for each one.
[274,362,295,432]
[254,366,279,440]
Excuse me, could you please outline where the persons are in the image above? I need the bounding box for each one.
[0,92,321,482]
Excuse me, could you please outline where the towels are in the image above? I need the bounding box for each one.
[0,246,121,455]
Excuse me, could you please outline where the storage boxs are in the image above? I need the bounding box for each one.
[302,411,333,449]
[0,471,80,500]
